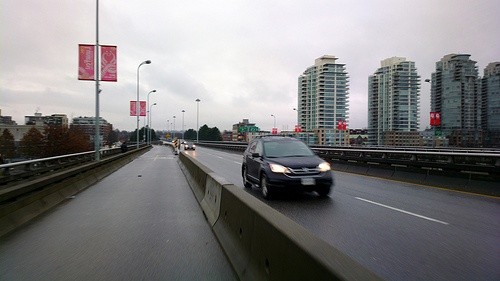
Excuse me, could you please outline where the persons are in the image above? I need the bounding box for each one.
[121,142,129,152]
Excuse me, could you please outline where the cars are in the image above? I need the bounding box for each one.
[167,138,195,150]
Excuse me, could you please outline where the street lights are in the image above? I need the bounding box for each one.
[181,110,185,142]
[196,99,200,143]
[173,115,176,138]
[425,79,436,149]
[271,114,275,128]
[136,60,151,149]
[146,89,157,146]
[150,103,157,145]
[293,108,299,128]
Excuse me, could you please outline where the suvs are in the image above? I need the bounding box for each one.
[241,134,333,201]
